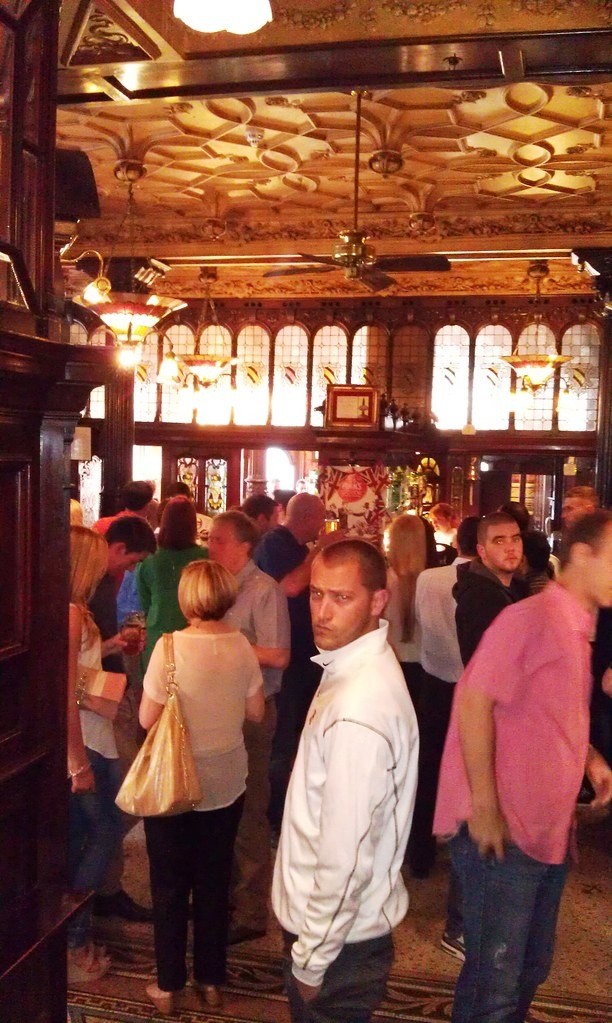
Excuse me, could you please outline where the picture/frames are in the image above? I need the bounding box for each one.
[324,383,380,431]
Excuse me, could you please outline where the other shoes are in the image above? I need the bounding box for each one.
[226,923,263,946]
[94,890,153,922]
[408,862,429,878]
[187,904,194,920]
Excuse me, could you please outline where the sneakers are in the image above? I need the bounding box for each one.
[440,930,467,962]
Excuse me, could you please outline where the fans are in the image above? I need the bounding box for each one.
[262,88,450,293]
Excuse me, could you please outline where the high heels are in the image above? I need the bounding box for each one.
[66,942,112,985]
[145,982,175,1016]
[189,972,222,1008]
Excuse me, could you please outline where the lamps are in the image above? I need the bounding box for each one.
[176,266,243,406]
[72,157,188,369]
[173,0,273,34]
[496,264,583,413]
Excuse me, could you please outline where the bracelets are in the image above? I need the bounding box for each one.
[70,761,90,777]
[313,544,324,551]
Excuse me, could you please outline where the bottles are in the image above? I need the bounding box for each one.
[120,610,147,664]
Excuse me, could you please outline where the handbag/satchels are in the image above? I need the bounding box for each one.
[74,663,127,719]
[115,633,202,818]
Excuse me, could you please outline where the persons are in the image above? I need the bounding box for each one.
[499,485,612,806]
[242,489,350,835]
[138,559,265,1015]
[384,503,457,686]
[431,510,612,1023]
[66,524,147,984]
[87,515,158,922]
[271,538,419,1023]
[410,516,481,878]
[440,513,535,962]
[69,481,297,673]
[210,511,292,946]
